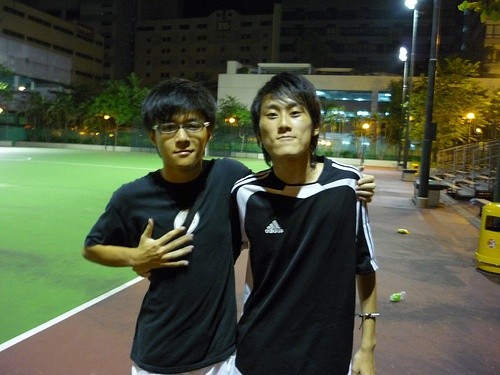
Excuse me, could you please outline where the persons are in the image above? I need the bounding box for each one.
[81,78,378,375]
[133,70,380,375]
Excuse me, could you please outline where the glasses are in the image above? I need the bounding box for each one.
[151,118,210,133]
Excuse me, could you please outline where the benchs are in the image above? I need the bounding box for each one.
[435,166,497,200]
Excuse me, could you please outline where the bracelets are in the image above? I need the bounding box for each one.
[356,312,380,329]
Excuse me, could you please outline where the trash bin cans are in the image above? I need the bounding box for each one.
[477,203,500,275]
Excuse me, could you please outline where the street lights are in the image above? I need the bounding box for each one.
[403,0,420,172]
[397,46,408,106]
